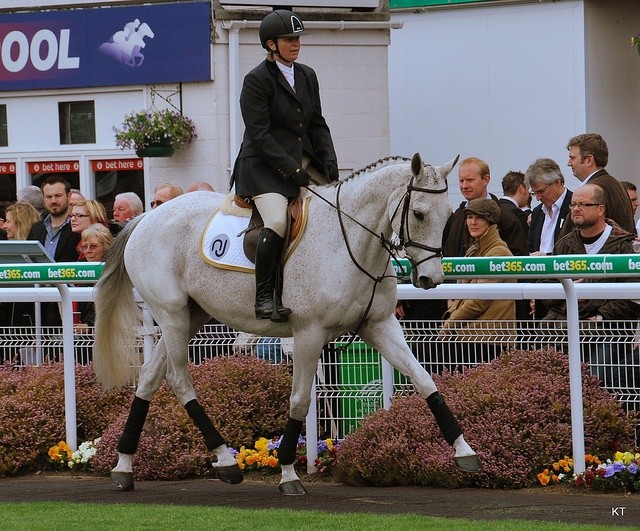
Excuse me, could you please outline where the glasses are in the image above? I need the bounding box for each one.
[81,245,101,249]
[569,203,601,209]
[68,212,90,218]
[533,185,550,196]
[150,200,163,208]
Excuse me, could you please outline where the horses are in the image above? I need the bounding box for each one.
[89,151,483,498]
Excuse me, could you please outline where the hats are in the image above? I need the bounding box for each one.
[461,198,501,224]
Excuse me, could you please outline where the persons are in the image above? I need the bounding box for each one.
[393,280,449,319]
[441,157,527,259]
[568,133,637,239]
[534,183,633,380]
[435,199,518,343]
[621,181,639,218]
[229,10,339,319]
[500,171,526,233]
[0,178,215,332]
[527,157,574,258]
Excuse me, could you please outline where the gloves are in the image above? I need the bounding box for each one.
[324,161,340,182]
[288,166,311,187]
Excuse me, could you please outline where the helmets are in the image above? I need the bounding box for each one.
[259,10,312,49]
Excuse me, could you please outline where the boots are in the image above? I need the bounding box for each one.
[255,228,292,320]
